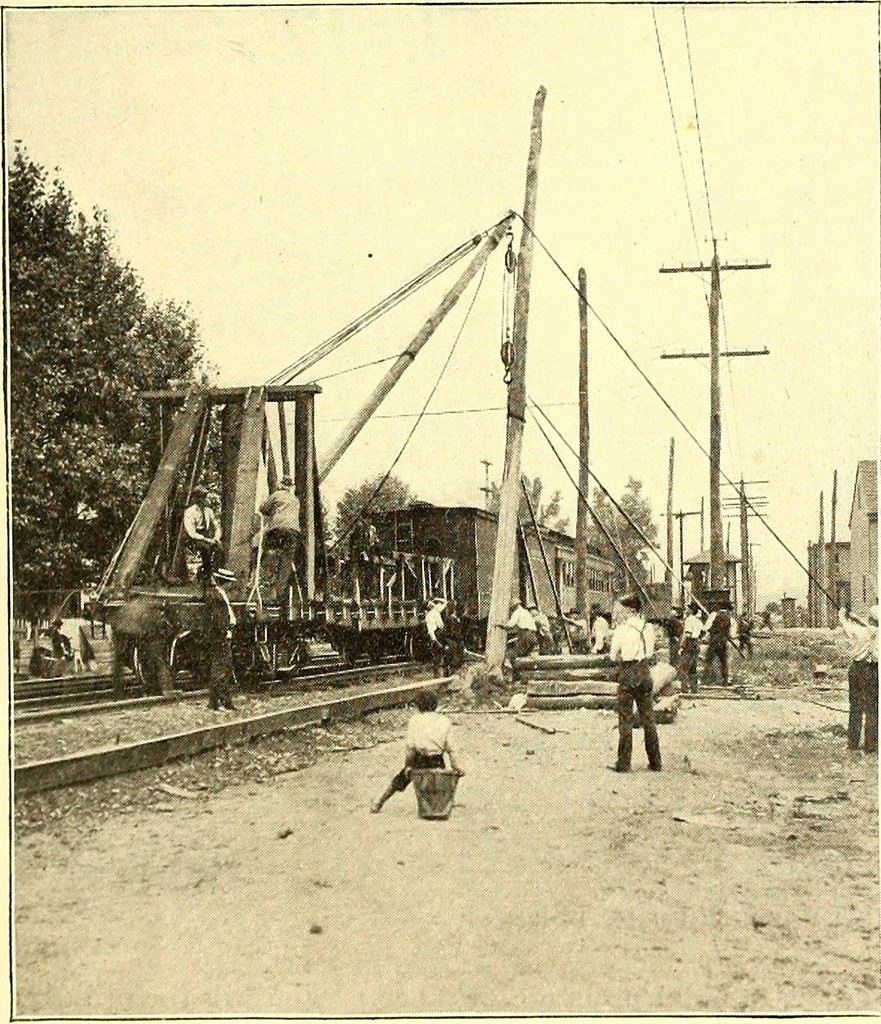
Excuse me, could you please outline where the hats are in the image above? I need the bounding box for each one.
[432,597,446,603]
[279,477,294,489]
[192,483,211,503]
[212,569,237,583]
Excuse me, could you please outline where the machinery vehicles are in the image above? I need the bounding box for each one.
[78,211,521,672]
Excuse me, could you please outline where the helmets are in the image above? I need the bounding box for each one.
[525,602,537,608]
[868,603,879,621]
[508,596,522,609]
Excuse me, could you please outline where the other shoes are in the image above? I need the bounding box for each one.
[607,760,618,773]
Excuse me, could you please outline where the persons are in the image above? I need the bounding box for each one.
[207,586,239,712]
[837,604,878,755]
[605,592,661,772]
[260,475,301,598]
[360,521,383,598]
[182,486,235,585]
[557,602,611,655]
[45,617,74,660]
[496,598,556,681]
[736,612,757,660]
[425,596,465,678]
[663,603,735,694]
[369,690,461,815]
